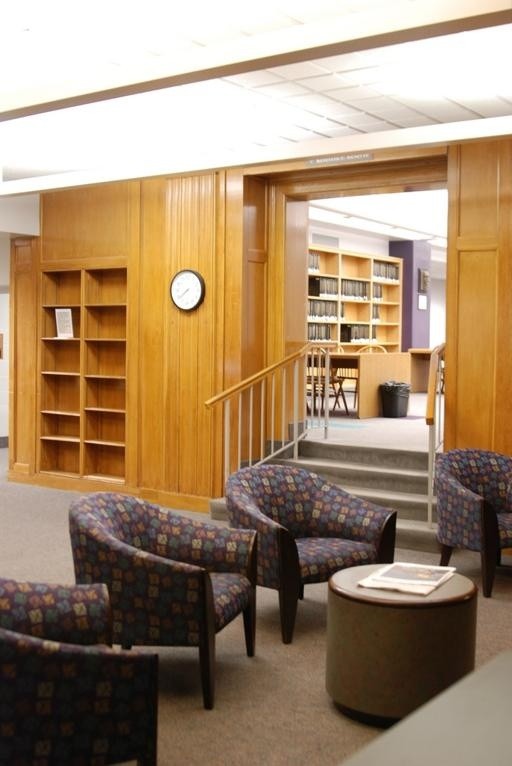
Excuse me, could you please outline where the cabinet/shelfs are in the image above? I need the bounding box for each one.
[38,263,125,487]
[306,245,404,389]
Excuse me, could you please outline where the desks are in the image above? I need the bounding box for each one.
[307,351,412,420]
[327,563,479,732]
[407,348,433,394]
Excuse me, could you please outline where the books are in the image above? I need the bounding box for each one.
[306,252,402,388]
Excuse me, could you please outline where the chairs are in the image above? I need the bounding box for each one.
[435,449,512,599]
[1,577,160,765]
[306,338,387,416]
[224,463,398,644]
[68,491,259,710]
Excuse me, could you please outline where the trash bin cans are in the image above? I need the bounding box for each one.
[378,381,410,417]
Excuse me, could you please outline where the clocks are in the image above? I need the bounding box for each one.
[168,270,205,310]
